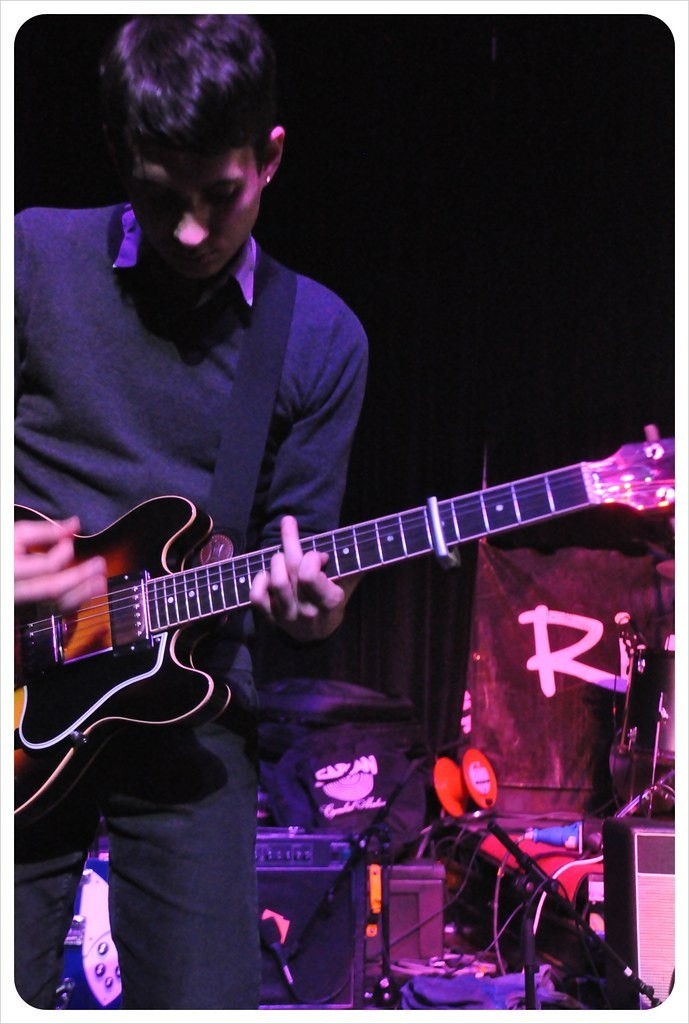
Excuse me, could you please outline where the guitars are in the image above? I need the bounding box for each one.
[14,425,673,836]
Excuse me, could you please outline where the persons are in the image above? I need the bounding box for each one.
[15,17,367,1010]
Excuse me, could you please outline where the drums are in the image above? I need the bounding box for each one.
[621,647,673,765]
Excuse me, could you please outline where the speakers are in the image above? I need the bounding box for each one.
[255,827,367,1010]
